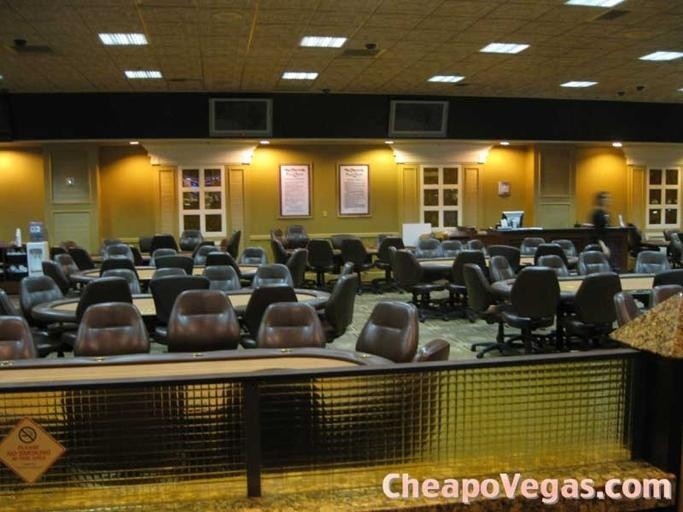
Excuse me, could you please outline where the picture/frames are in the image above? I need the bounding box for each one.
[278,161,312,220]
[336,163,373,218]
[497,181,511,197]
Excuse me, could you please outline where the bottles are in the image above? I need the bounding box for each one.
[15,228,23,247]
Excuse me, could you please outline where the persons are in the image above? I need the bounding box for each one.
[589,191,612,267]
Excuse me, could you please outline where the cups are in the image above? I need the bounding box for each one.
[511,220,517,228]
